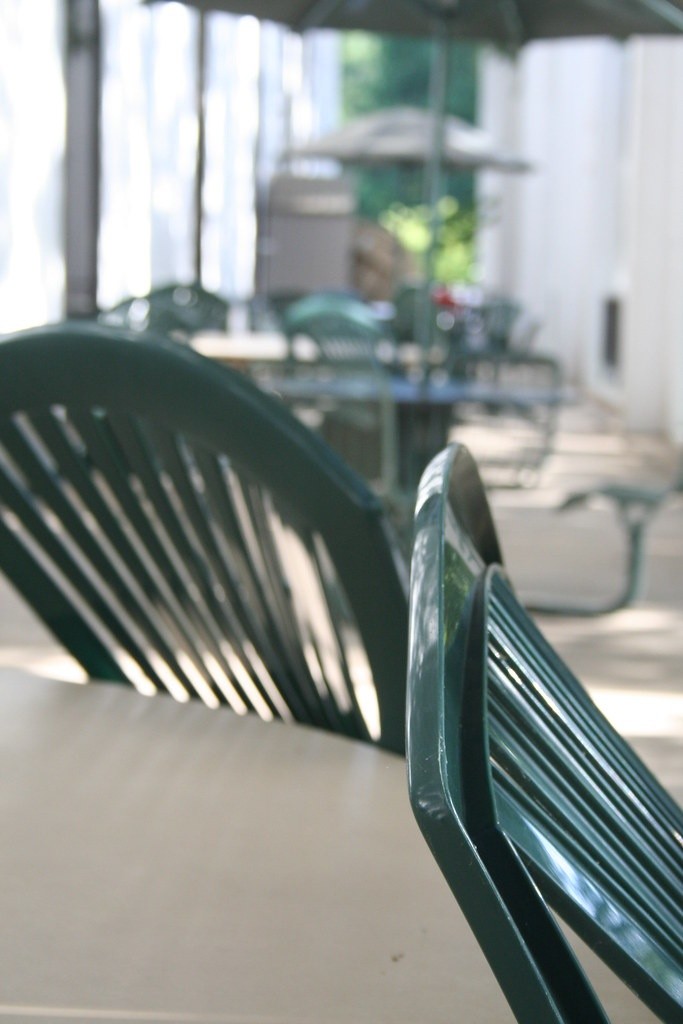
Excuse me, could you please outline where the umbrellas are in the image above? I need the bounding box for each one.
[141,0,680,384]
[291,112,534,176]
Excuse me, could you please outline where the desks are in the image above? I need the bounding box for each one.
[0,667,676,1024]
[188,330,443,386]
[256,372,575,497]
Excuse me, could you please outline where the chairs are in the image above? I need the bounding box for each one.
[406,444,682,1024]
[0,321,415,758]
